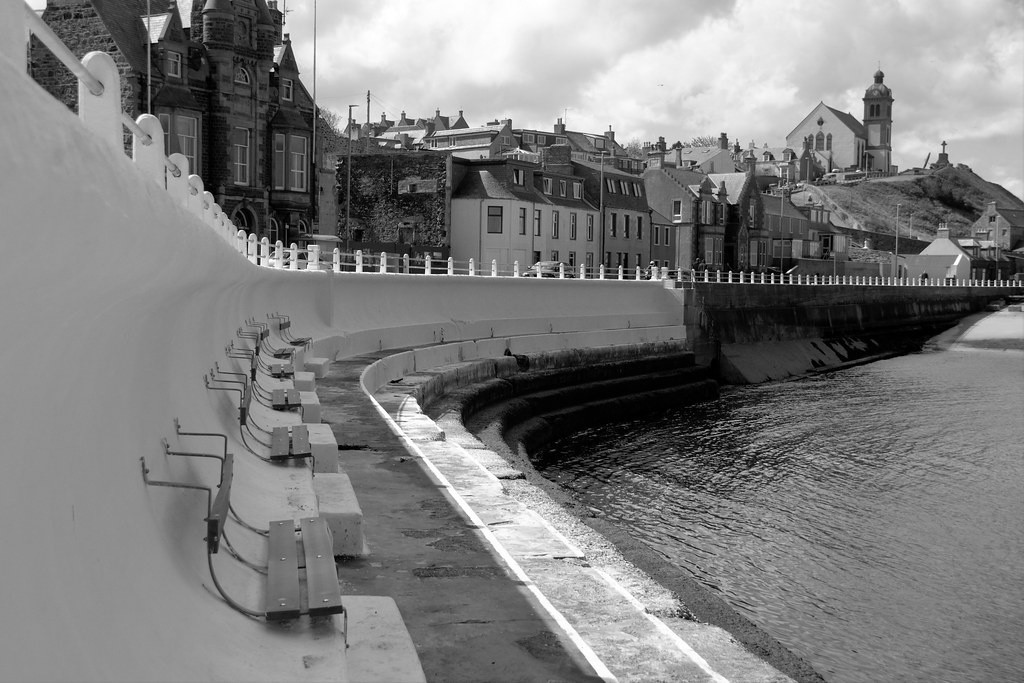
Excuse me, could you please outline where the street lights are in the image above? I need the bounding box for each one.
[895,203,903,278]
[599,150,611,264]
[346,104,360,254]
[996,216,1002,282]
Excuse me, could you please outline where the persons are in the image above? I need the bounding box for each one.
[646,261,656,279]
[692,258,707,281]
[921,270,928,285]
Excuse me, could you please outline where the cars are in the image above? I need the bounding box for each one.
[522,260,575,279]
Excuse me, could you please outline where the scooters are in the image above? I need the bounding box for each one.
[643,269,653,280]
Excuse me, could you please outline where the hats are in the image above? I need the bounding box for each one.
[701,259,706,262]
[650,261,654,264]
